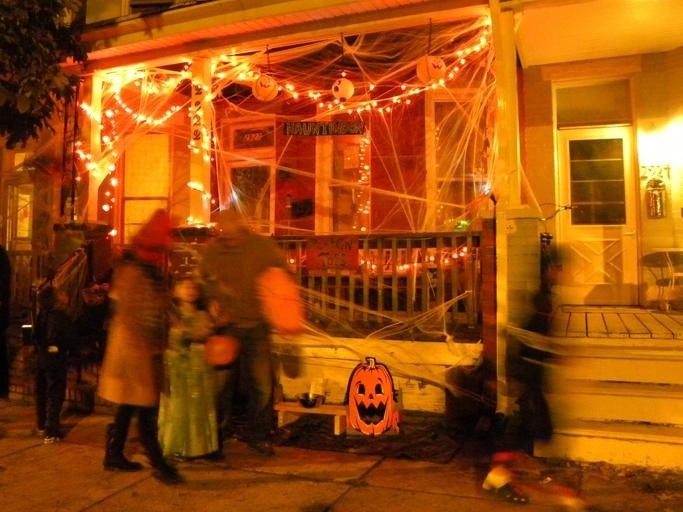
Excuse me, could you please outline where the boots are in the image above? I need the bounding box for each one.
[101,422,145,471]
[140,429,179,476]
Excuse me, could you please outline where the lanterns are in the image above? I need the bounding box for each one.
[332,77,354,101]
[416,55,446,83]
[252,74,278,102]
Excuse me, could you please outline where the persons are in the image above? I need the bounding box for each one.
[97,212,297,484]
[32,286,77,445]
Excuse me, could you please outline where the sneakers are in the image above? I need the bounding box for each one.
[34,426,61,445]
